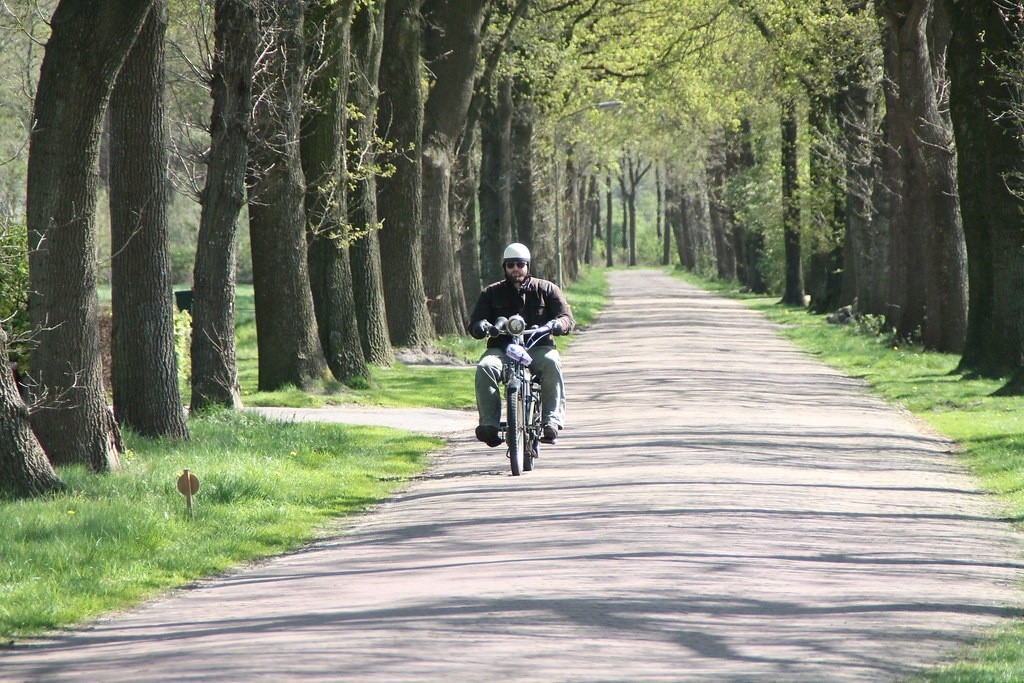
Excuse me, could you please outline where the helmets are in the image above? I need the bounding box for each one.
[504,243,531,264]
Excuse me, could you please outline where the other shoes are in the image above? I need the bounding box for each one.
[543,421,559,442]
[475,424,501,449]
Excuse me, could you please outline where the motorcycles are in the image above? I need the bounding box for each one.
[483,314,558,478]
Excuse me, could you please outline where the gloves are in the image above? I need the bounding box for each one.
[550,318,563,336]
[476,320,492,339]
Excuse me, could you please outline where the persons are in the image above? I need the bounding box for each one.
[468,242,575,449]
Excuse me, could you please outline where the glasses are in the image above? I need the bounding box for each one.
[506,262,526,270]
